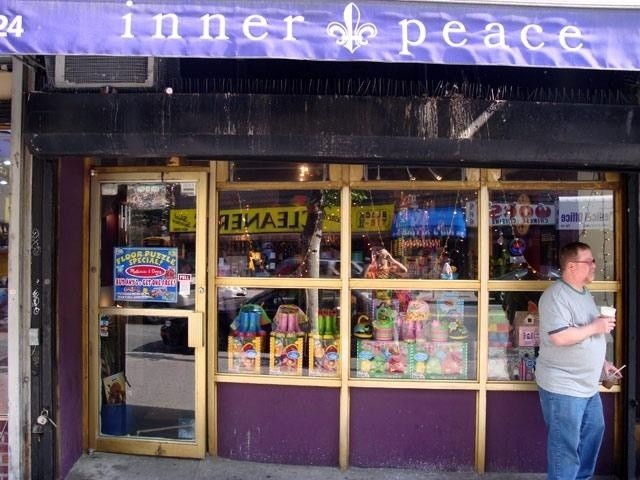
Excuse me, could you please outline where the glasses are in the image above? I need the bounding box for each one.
[573,258,596,265]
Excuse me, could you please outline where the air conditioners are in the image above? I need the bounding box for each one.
[53,56,162,91]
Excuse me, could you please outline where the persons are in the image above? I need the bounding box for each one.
[364,239,454,280]
[535,242,623,480]
